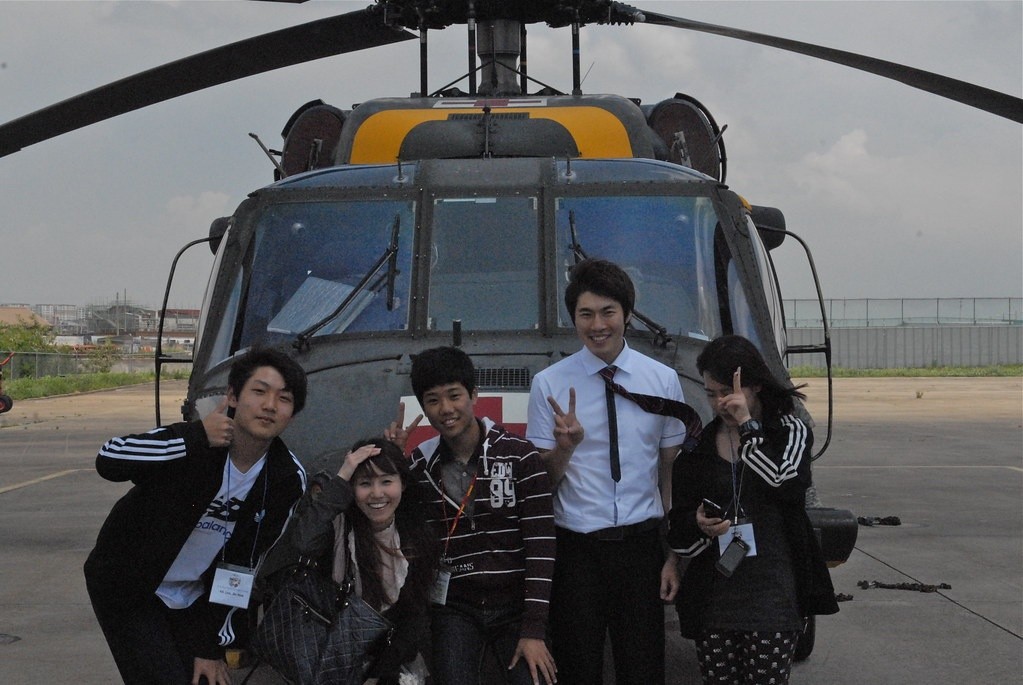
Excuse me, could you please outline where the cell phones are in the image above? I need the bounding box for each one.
[714,536,750,577]
[702,498,723,519]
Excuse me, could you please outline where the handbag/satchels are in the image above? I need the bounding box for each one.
[248,499,396,685]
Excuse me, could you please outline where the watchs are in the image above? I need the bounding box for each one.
[737,418,762,437]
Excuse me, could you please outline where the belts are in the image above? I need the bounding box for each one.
[555,518,661,541]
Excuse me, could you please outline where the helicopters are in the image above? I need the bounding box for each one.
[1,1,1023,665]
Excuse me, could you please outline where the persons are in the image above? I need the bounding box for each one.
[526,258,688,684]
[383,344,559,685]
[290,438,433,685]
[665,333,841,685]
[82,348,310,685]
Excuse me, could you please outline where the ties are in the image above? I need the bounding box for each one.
[597,366,703,483]
